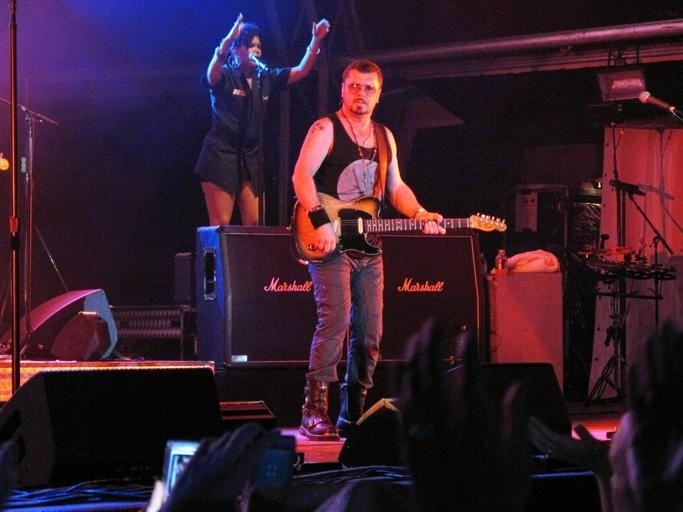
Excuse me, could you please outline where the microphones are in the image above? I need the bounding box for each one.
[248,52,270,72]
[610,180,644,195]
[639,91,675,113]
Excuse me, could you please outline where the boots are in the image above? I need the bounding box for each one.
[341,381,367,432]
[301,377,338,439]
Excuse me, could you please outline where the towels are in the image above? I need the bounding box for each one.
[504,247,560,274]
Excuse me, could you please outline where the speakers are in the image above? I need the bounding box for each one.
[376,230,488,365]
[401,362,572,453]
[0,369,224,491]
[1,288,118,361]
[197,225,348,367]
[340,396,405,469]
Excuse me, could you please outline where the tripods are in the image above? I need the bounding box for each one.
[584,272,642,408]
[0,97,61,361]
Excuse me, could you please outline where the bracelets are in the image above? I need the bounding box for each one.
[305,203,331,230]
[307,43,320,56]
[409,207,425,220]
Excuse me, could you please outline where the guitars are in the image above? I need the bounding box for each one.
[292,190,510,265]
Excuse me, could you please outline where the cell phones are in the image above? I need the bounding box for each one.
[162,440,201,509]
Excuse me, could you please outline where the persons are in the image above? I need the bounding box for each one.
[191,12,331,230]
[290,57,447,441]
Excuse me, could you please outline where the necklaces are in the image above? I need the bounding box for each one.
[339,103,377,168]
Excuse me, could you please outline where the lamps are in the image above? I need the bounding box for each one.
[596,41,647,104]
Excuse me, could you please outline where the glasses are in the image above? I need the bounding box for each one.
[345,80,378,96]
